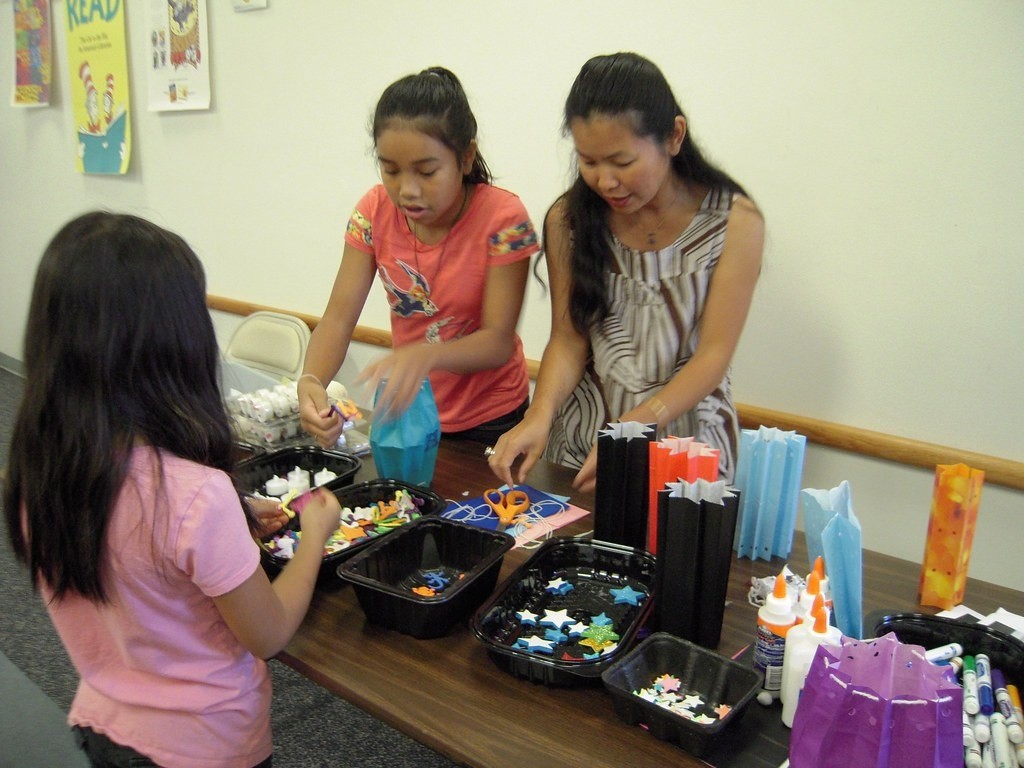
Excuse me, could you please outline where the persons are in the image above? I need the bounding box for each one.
[295,66,539,451]
[5,210,344,768]
[487,53,766,496]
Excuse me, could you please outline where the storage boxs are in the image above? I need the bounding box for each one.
[468,535,668,690]
[337,514,515,640]
[253,477,448,604]
[225,442,363,512]
[600,630,766,758]
[870,611,1024,699]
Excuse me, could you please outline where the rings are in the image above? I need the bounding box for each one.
[313,432,318,441]
[484,446,496,457]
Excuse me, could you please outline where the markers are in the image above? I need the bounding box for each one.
[922,643,1024,767]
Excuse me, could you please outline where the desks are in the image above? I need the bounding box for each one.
[211,395,1023,767]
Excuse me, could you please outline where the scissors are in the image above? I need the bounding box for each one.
[484,487,531,531]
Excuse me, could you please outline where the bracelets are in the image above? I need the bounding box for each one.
[637,393,668,435]
[299,374,326,388]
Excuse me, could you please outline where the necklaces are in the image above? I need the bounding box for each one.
[412,182,470,297]
[626,169,682,246]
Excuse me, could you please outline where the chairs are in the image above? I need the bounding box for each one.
[224,310,313,385]
